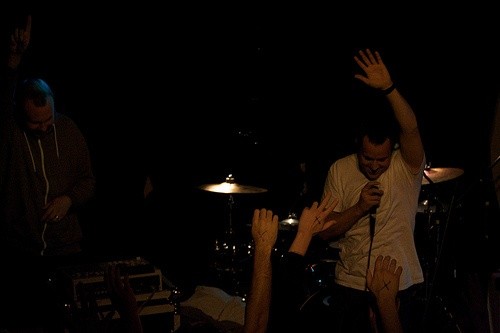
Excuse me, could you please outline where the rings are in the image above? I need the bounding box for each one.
[55,215,60,221]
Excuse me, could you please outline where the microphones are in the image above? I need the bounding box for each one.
[369,185,378,240]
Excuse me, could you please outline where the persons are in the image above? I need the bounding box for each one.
[173,192,402,333]
[104,263,144,333]
[313,49,426,333]
[461,273,488,333]
[0,15,95,333]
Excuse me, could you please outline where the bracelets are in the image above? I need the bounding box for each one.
[382,84,396,96]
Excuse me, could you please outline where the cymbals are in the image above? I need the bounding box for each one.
[420,161,464,188]
[197,178,268,196]
